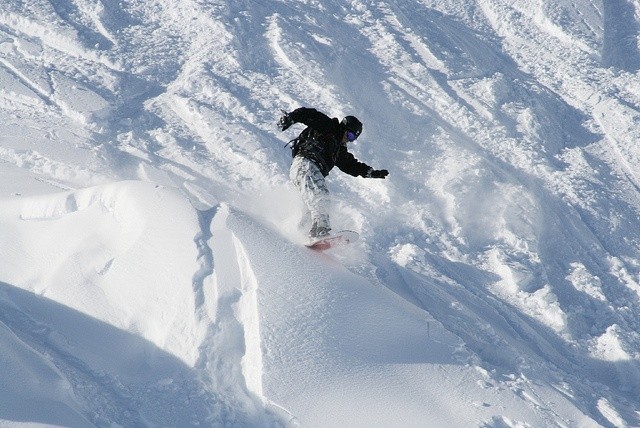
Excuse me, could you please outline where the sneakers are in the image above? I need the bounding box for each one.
[308,225,317,240]
[315,227,331,238]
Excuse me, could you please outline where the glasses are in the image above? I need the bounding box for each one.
[346,130,357,142]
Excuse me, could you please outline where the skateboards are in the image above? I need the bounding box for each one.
[304,230,359,249]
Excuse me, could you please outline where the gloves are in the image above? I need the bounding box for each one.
[276,115,292,132]
[370,169,389,179]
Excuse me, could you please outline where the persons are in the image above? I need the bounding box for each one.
[276,106,389,238]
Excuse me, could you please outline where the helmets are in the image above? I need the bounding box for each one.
[341,115,363,137]
